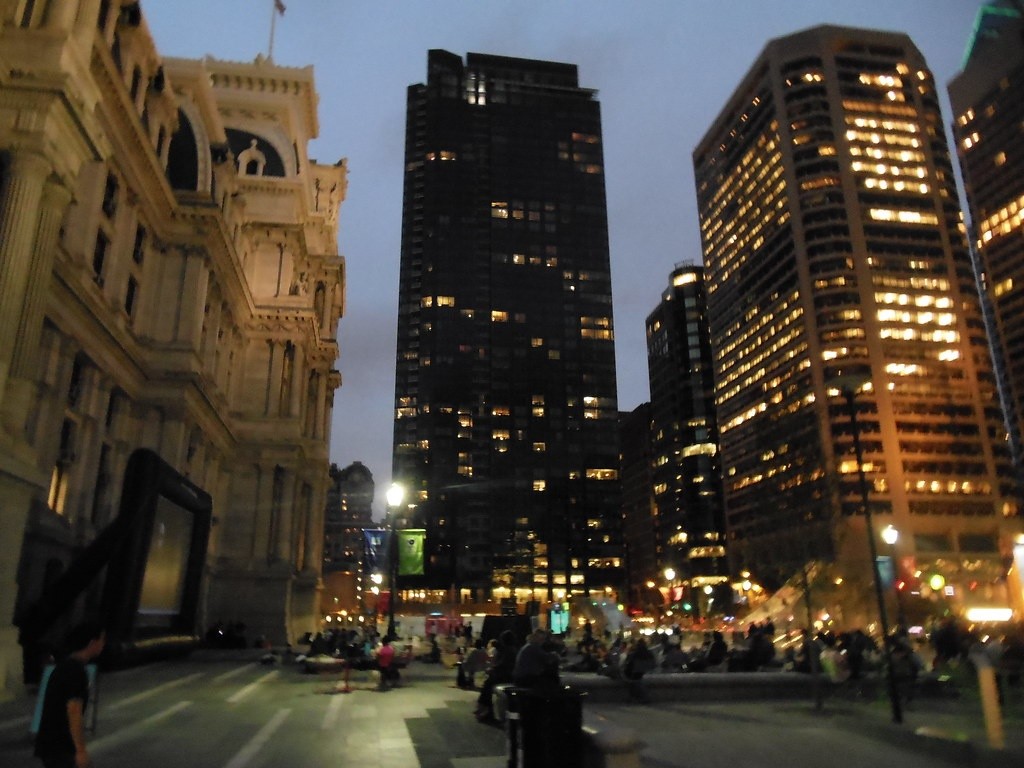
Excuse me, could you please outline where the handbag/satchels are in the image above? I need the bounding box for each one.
[635,660,652,679]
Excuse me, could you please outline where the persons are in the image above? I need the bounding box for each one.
[30,614,105,768]
[254,634,272,649]
[290,591,1022,768]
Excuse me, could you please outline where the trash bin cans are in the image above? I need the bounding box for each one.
[502,685,589,768]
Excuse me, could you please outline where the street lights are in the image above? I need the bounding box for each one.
[823,361,905,726]
[878,524,906,621]
[664,569,676,630]
[382,482,405,636]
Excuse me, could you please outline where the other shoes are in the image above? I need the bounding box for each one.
[474,710,496,721]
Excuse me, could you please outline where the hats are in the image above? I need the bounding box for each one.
[526,628,546,643]
